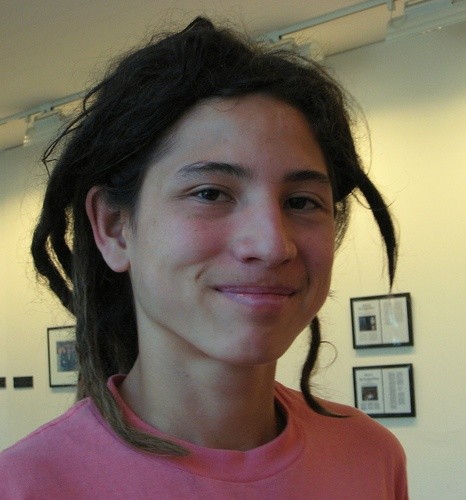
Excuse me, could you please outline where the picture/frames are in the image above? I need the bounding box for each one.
[353,364,416,419]
[47,324,80,388]
[349,293,414,349]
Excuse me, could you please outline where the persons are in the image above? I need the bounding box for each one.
[0,15,408,500]
